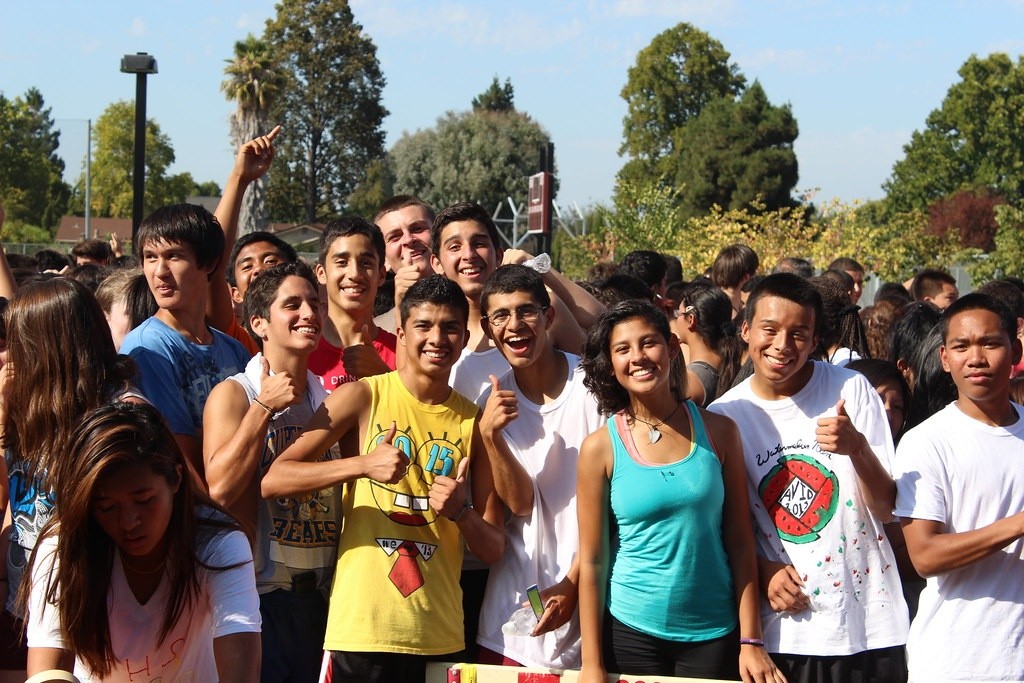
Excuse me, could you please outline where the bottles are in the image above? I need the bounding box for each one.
[522,252,552,273]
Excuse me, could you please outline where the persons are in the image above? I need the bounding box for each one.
[202,261,343,683]
[704,274,896,683]
[263,274,504,683]
[1,277,155,670]
[891,293,1024,683]
[0,125,1024,503]
[473,264,612,670]
[25,400,262,683]
[576,299,788,683]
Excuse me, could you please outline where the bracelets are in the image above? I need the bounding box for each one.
[252,398,273,416]
[740,639,764,646]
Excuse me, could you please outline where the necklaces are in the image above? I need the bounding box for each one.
[627,400,680,444]
[122,555,167,574]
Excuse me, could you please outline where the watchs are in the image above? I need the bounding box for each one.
[449,500,472,522]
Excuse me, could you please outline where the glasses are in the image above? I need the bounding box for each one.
[674,310,689,318]
[484,306,548,322]
